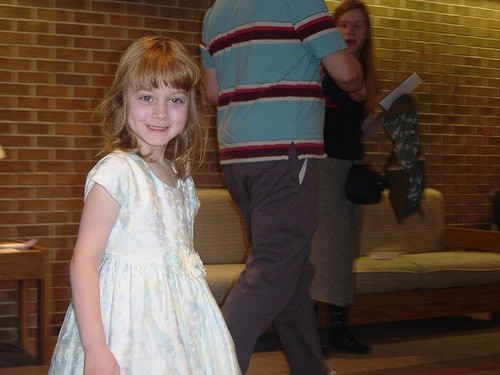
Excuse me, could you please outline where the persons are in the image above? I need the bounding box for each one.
[199,0,370,375]
[309,0,414,362]
[47,37,242,375]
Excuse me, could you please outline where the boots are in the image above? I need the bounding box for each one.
[328,303,369,353]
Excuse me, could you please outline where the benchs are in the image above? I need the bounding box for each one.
[193,188,500,361]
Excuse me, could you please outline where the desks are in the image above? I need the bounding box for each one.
[0,240,50,375]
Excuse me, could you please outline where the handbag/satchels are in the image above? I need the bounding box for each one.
[345,164,381,205]
